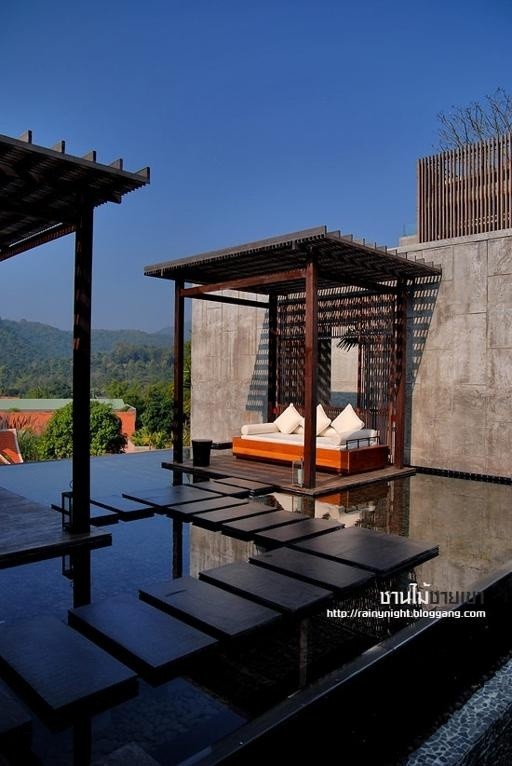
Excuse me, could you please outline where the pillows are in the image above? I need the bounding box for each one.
[240,405,377,445]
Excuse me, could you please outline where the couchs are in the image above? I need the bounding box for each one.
[231,433,388,474]
